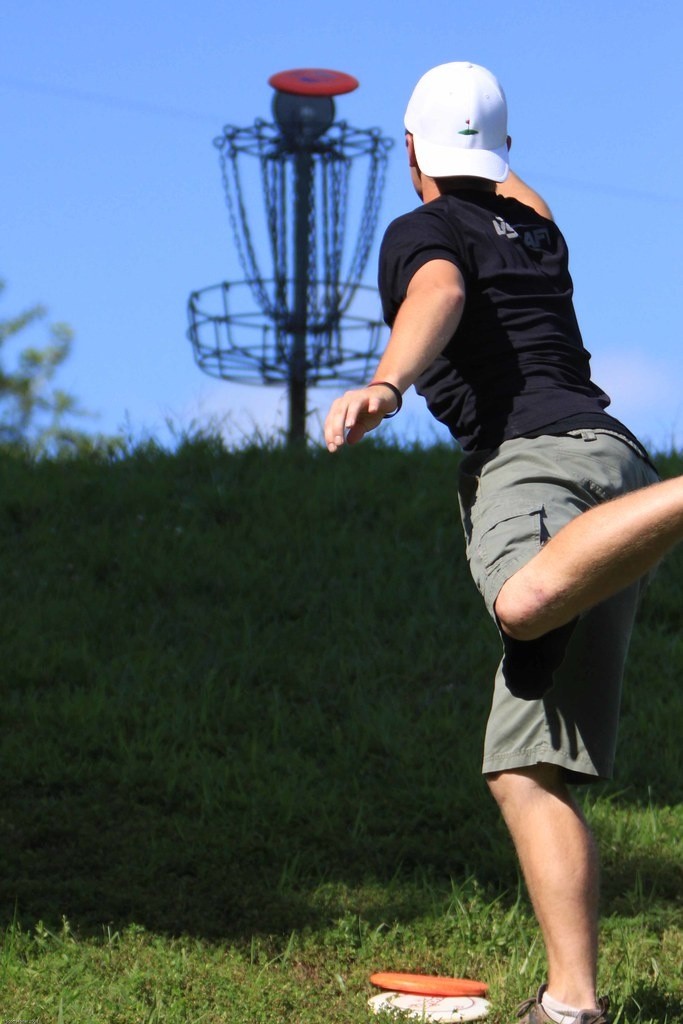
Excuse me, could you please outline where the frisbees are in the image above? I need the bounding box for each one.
[369,992,493,1023]
[370,971,488,997]
[269,67,361,97]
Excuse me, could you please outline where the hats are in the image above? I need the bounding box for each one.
[404,62,510,183]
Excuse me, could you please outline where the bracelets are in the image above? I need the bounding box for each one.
[368,382,404,419]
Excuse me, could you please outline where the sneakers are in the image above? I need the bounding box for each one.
[504,985,604,1024]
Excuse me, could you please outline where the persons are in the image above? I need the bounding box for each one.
[321,61,683,1024]
